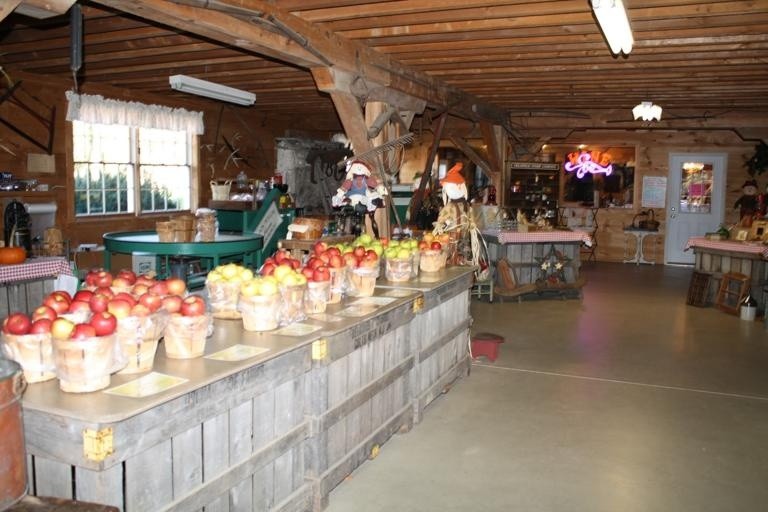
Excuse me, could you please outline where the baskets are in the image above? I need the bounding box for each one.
[644,209,659,230]
[209,180,232,200]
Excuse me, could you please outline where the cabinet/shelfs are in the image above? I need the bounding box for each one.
[20,265,476,512]
[481,229,592,303]
[684,237,768,316]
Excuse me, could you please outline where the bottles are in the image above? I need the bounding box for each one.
[228,171,293,210]
[327,207,377,238]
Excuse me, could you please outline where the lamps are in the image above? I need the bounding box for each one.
[591,0,634,55]
[632,101,662,123]
[169,74,257,106]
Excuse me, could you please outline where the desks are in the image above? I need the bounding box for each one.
[278,235,356,254]
[103,231,263,291]
[623,228,658,267]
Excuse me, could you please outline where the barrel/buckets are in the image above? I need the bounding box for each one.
[0,180,448,395]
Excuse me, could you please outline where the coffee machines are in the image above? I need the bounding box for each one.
[2,197,34,258]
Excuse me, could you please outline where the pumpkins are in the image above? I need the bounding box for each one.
[0,247,26,264]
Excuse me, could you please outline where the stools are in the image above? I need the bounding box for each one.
[471,281,493,304]
[471,333,504,361]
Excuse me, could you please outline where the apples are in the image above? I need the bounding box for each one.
[2,271,206,341]
[207,232,449,297]
